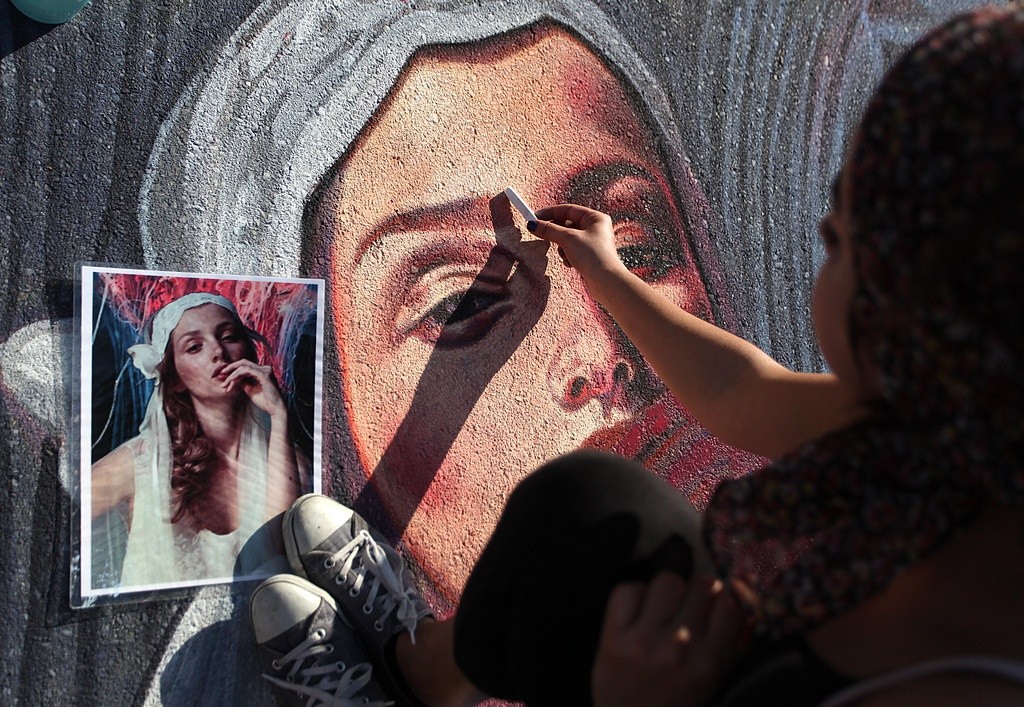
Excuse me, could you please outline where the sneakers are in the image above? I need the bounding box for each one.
[283,493,437,707]
[250,574,411,707]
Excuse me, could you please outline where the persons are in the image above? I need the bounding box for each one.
[243,0,1024,707]
[90,293,314,589]
